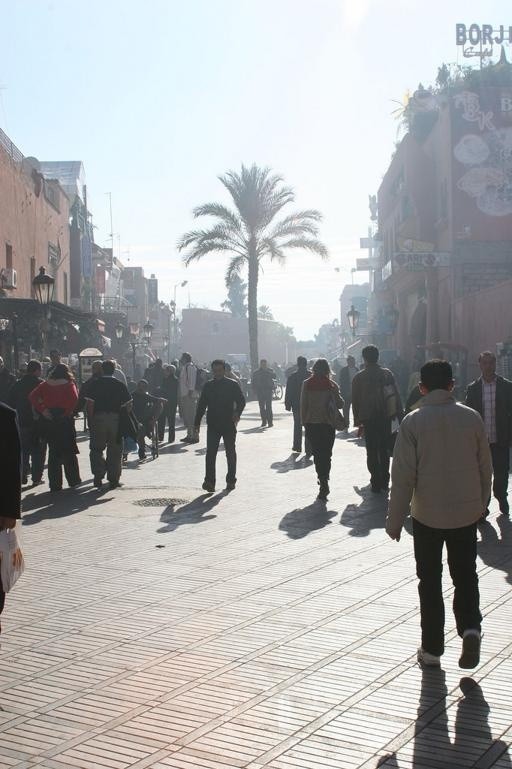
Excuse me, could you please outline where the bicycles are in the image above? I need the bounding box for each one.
[270,378,284,400]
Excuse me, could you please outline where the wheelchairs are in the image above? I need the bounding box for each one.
[121,419,162,465]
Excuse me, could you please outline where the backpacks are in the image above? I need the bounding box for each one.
[376,371,399,417]
[186,363,210,390]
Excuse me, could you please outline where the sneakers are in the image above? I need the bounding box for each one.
[202,482,214,492]
[495,494,509,513]
[459,629,481,669]
[417,648,440,666]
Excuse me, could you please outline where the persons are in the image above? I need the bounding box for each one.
[299,358,345,498]
[123,379,168,463]
[329,355,360,432]
[194,359,246,493]
[272,361,283,384]
[48,348,61,374]
[285,357,313,456]
[463,351,512,519]
[251,358,277,427]
[352,345,403,493]
[80,359,133,490]
[386,358,493,669]
[144,351,212,444]
[4,359,47,487]
[405,386,424,416]
[0,401,23,634]
[29,363,81,492]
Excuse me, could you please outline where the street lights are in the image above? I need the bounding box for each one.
[158,298,176,358]
[110,317,157,381]
[160,332,171,362]
[338,329,348,352]
[30,262,60,367]
[173,279,188,360]
[345,303,362,341]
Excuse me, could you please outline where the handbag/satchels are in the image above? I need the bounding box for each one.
[328,398,346,430]
[0,529,24,593]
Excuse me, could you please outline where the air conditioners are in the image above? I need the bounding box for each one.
[3,268,18,289]
